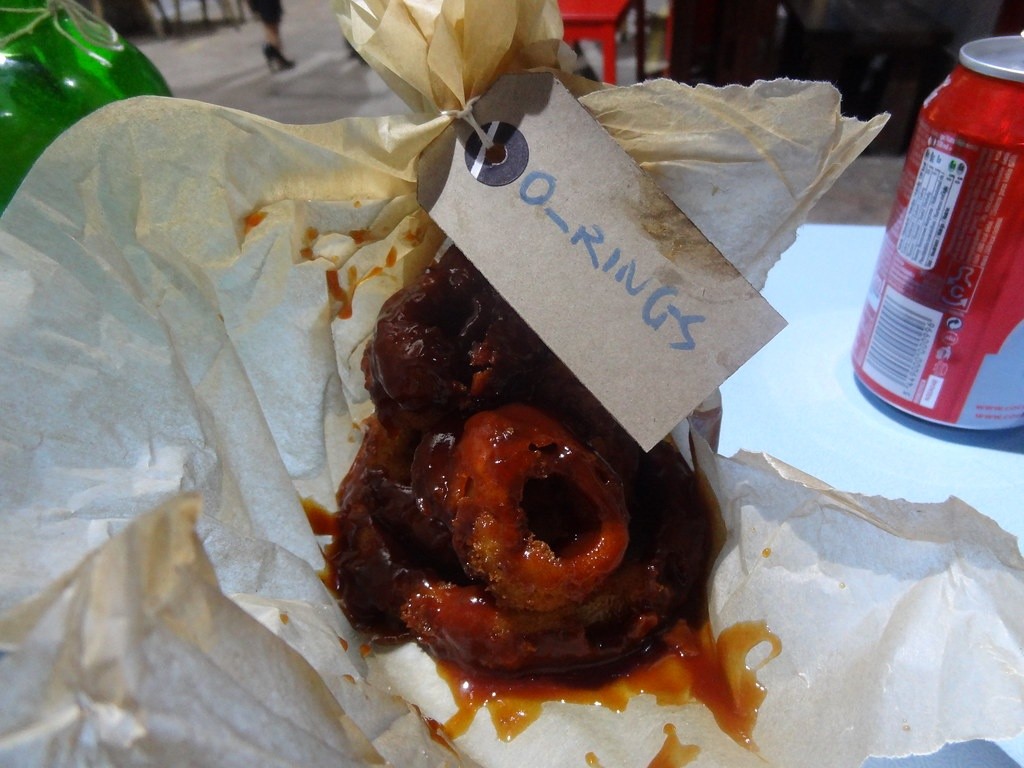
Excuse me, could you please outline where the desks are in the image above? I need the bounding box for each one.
[779,0,957,154]
[718,153,1024,768]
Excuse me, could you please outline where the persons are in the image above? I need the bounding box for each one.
[244,0,295,72]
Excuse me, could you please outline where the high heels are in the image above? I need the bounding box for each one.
[262,42,295,72]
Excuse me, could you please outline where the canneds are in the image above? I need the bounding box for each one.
[852,31,1024,432]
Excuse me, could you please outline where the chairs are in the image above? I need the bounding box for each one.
[557,0,645,86]
